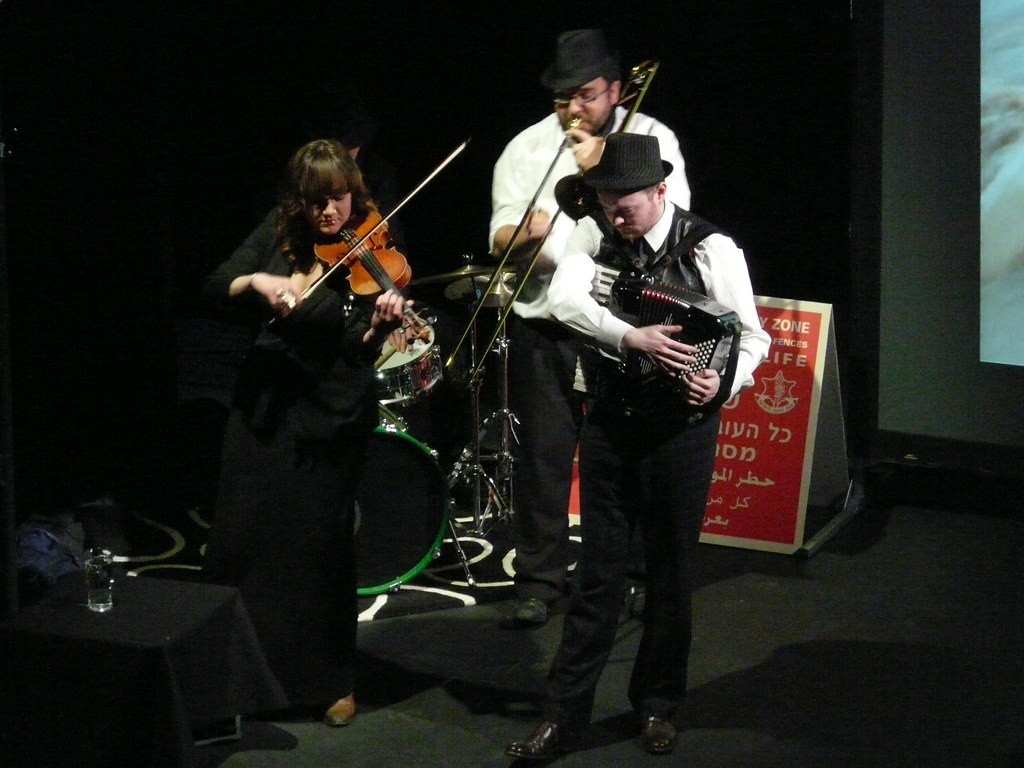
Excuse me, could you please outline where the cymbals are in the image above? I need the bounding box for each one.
[407,264,517,286]
[444,272,543,308]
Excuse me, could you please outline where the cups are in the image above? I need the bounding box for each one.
[84,547,114,612]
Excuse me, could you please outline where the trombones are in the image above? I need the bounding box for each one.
[443,54,662,388]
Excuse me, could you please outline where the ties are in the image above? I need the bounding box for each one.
[634,237,642,258]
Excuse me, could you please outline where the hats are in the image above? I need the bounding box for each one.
[584,132,674,190]
[541,28,623,93]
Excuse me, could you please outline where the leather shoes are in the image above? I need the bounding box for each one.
[505,722,578,760]
[323,692,356,726]
[641,716,676,752]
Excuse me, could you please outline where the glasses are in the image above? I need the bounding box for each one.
[552,85,612,104]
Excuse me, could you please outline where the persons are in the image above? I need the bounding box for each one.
[206,141,415,724]
[489,30,690,622]
[504,133,772,760]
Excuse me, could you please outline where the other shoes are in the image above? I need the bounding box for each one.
[516,598,547,625]
[625,587,644,614]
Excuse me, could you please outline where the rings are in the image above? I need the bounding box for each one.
[277,290,284,295]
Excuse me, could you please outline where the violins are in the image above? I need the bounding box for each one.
[313,206,438,345]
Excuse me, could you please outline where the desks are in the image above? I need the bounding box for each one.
[0,570,290,768]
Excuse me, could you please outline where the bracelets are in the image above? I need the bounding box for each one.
[249,273,256,287]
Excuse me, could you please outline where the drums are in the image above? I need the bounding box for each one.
[374,316,444,409]
[351,401,454,599]
